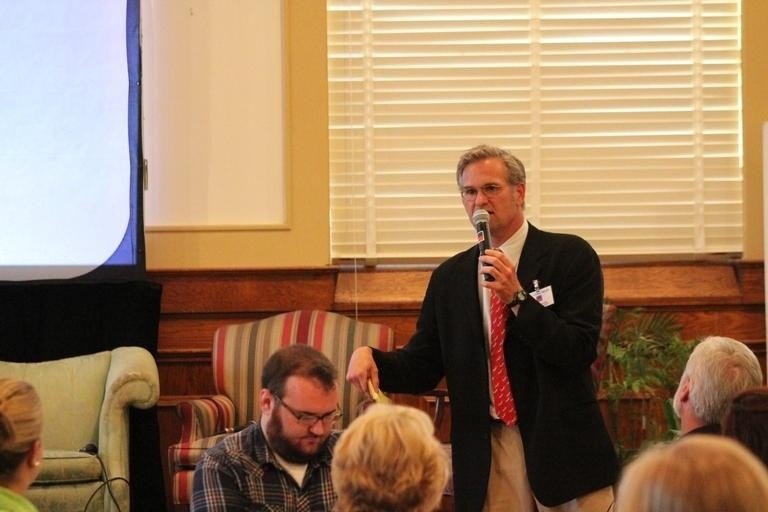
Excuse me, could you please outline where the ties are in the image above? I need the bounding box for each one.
[489,289,517,427]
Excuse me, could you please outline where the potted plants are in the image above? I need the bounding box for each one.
[589,308,705,465]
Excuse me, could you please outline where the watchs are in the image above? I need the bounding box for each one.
[506,290,526,308]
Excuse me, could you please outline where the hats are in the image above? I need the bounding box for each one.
[0,379,42,453]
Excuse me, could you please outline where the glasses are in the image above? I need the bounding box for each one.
[461,185,505,200]
[273,394,343,425]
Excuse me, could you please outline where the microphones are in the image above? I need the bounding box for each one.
[472,209,496,281]
[224,418,257,433]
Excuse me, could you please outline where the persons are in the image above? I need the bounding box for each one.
[0,378,44,512]
[618,336,768,511]
[190,344,347,512]
[347,145,617,512]
[331,404,450,512]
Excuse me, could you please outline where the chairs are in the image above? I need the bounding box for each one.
[0,346,160,512]
[167,310,394,505]
